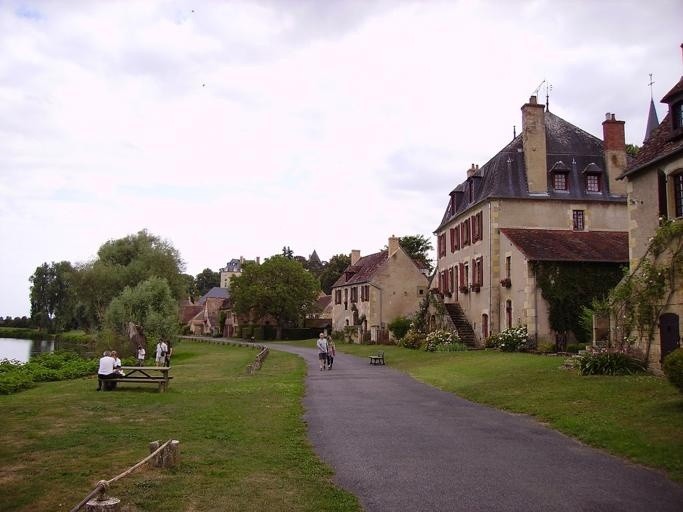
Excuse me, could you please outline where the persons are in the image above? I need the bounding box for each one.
[316,332,328,374]
[155,338,173,367]
[326,336,335,370]
[96,350,122,392]
[137,345,146,366]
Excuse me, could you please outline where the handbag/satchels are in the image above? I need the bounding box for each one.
[167,356,171,361]
[114,370,122,378]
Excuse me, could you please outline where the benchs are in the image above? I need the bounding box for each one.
[97,375,173,393]
[369,352,385,366]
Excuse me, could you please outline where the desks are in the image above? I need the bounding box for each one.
[114,367,171,393]
